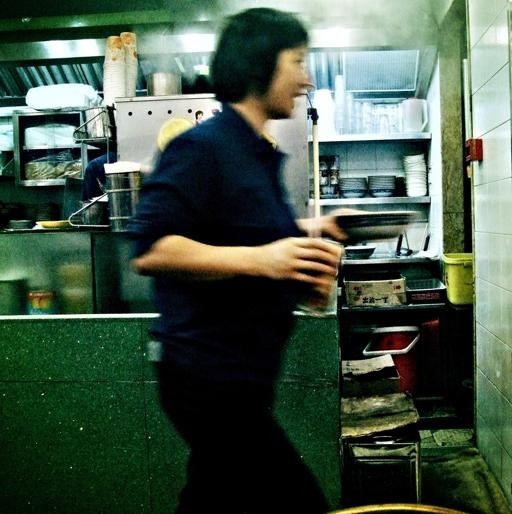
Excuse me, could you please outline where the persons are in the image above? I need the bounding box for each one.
[79,153,117,314]
[125,5,364,514]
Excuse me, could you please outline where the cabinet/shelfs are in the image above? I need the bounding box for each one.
[309,97,446,310]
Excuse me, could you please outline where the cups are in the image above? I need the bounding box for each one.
[402,99,428,134]
[335,75,345,135]
[345,92,354,135]
[313,89,334,136]
[120,32,138,97]
[354,102,361,134]
[103,36,126,104]
[361,102,402,135]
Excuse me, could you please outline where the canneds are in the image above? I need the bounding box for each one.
[26,293,53,316]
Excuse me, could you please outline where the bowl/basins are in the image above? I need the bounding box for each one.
[344,245,376,259]
[335,213,419,242]
[368,176,396,197]
[402,154,427,197]
[338,178,368,198]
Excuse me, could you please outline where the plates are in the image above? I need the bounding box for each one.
[36,220,69,227]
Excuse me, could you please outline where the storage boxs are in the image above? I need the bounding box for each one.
[343,274,407,306]
[440,253,473,305]
